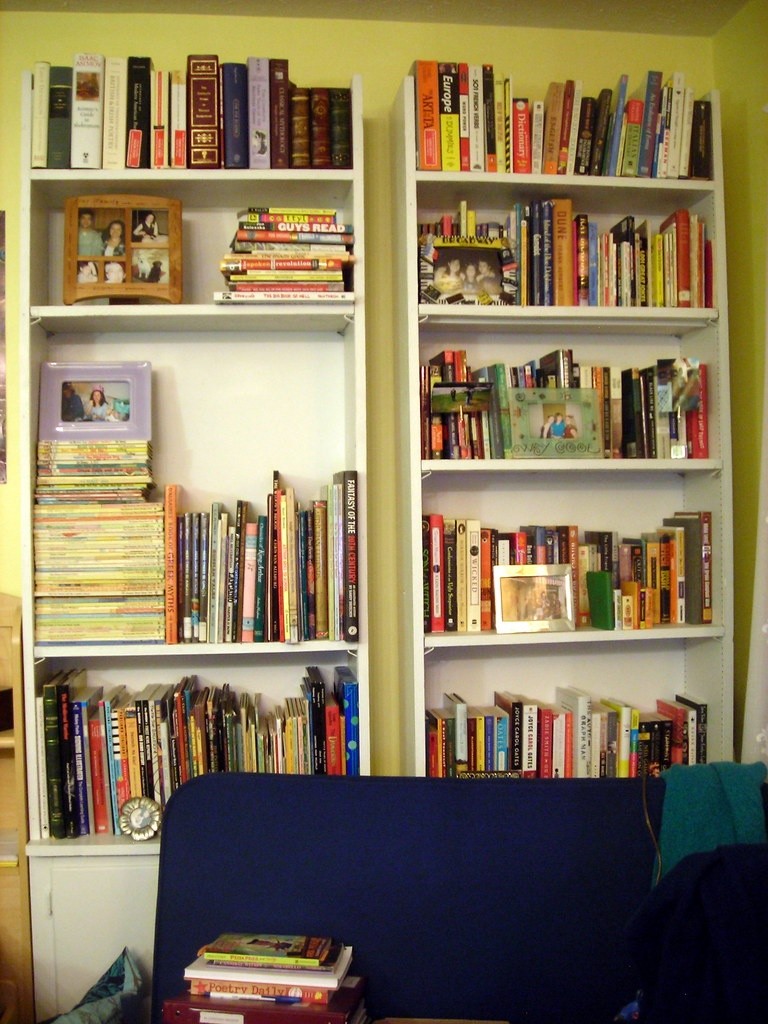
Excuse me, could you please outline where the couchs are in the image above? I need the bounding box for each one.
[147,762,766,1024]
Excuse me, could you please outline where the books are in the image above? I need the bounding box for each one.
[34,664,360,835]
[426,684,708,780]
[504,196,714,387]
[33,436,361,644]
[543,508,714,632]
[408,59,713,238]
[161,932,369,1024]
[212,206,357,303]
[417,348,525,632]
[590,346,710,460]
[28,52,353,171]
[519,524,547,564]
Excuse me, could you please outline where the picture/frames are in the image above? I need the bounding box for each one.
[64,194,182,304]
[508,387,604,460]
[38,360,153,442]
[492,564,575,633]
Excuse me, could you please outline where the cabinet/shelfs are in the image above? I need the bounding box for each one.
[22,70,367,1023]
[390,77,735,781]
[1,592,35,1024]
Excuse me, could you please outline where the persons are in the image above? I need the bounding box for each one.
[538,411,578,438]
[521,589,562,620]
[62,381,131,423]
[432,255,502,294]
[77,209,166,285]
